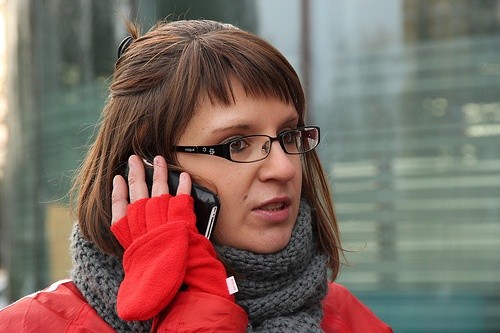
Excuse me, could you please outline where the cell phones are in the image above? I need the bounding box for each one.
[119,156,221,240]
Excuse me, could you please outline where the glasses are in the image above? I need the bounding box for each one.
[168,125,321,163]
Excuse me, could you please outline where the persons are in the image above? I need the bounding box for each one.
[0,6,393,333]
